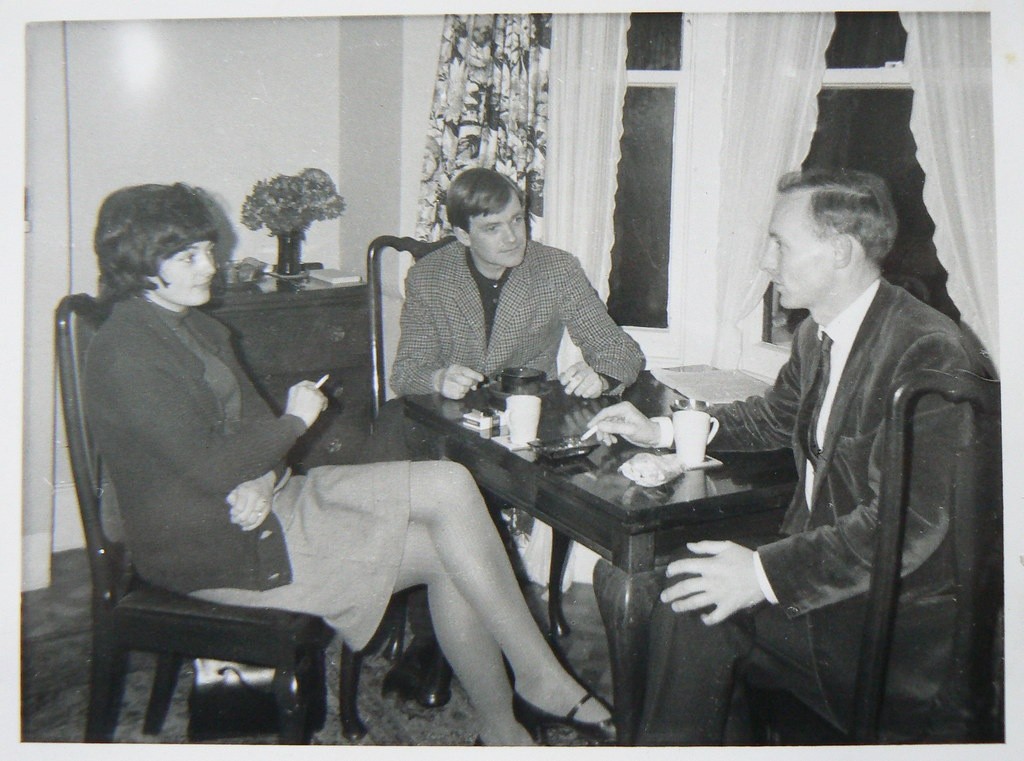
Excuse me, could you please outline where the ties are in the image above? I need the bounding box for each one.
[799,333,832,468]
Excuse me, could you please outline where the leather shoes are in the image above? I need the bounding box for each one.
[417,654,453,709]
[380,637,434,703]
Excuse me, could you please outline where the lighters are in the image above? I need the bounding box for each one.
[472,408,493,417]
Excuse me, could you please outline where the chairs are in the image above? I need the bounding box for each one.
[50,288,372,745]
[369,233,573,668]
[743,365,1003,746]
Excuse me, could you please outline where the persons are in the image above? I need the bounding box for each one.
[84,182,615,746]
[375,167,644,461]
[587,166,1005,747]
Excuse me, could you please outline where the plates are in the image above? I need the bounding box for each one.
[488,381,554,401]
[526,433,602,460]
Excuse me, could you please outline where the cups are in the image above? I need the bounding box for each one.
[495,366,543,395]
[672,410,720,466]
[504,395,542,445]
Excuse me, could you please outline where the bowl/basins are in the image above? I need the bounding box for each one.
[669,399,711,412]
[214,260,268,284]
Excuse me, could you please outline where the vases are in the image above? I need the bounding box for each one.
[270,229,311,276]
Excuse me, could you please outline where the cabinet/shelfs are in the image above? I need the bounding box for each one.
[194,278,372,477]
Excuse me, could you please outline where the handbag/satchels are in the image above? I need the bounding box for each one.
[187,655,300,743]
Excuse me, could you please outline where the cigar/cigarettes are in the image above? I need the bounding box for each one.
[315,375,329,388]
[581,426,597,441]
[569,377,577,382]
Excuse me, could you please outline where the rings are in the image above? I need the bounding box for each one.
[253,510,262,516]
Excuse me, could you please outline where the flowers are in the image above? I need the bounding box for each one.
[238,166,348,238]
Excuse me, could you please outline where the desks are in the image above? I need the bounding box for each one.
[398,362,800,746]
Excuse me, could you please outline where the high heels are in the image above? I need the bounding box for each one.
[473,734,487,747]
[513,678,617,741]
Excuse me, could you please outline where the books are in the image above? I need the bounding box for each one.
[309,268,360,283]
